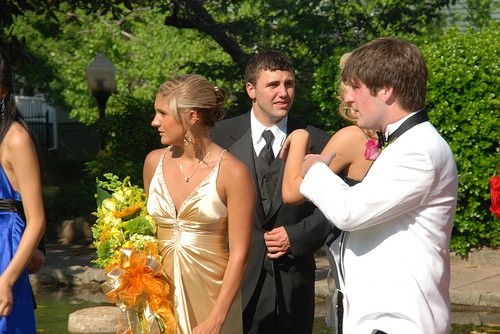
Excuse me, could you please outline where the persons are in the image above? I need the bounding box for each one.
[0,76,46,334]
[301,37,458,334]
[209,50,345,334]
[280,52,381,334]
[143,74,256,334]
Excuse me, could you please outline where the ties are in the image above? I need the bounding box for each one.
[259,130,275,180]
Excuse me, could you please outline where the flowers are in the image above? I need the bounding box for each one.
[91,173,183,334]
[365,138,382,160]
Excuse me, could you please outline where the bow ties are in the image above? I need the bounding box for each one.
[375,131,386,148]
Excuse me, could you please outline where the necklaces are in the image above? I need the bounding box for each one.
[177,142,215,182]
